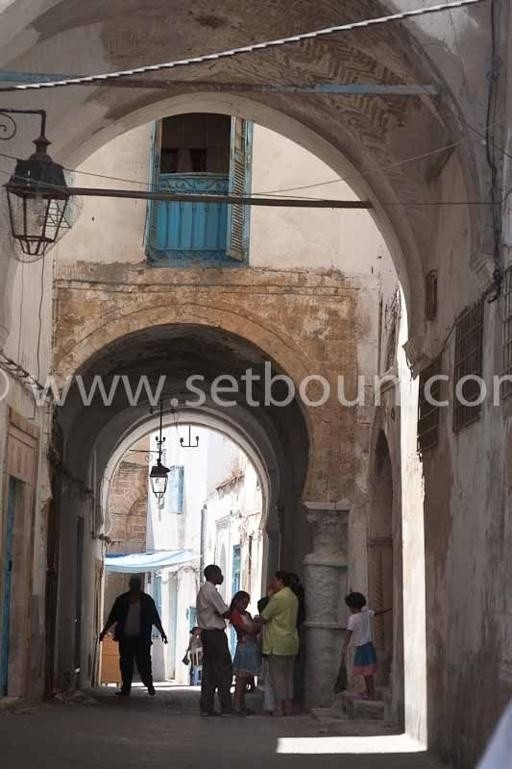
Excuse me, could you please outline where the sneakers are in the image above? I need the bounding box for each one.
[115,686,256,718]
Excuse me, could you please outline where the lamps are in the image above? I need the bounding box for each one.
[149,399,171,498]
[0,108,70,256]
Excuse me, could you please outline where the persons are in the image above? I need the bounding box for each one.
[99,574,167,696]
[187,565,305,717]
[342,592,392,700]
